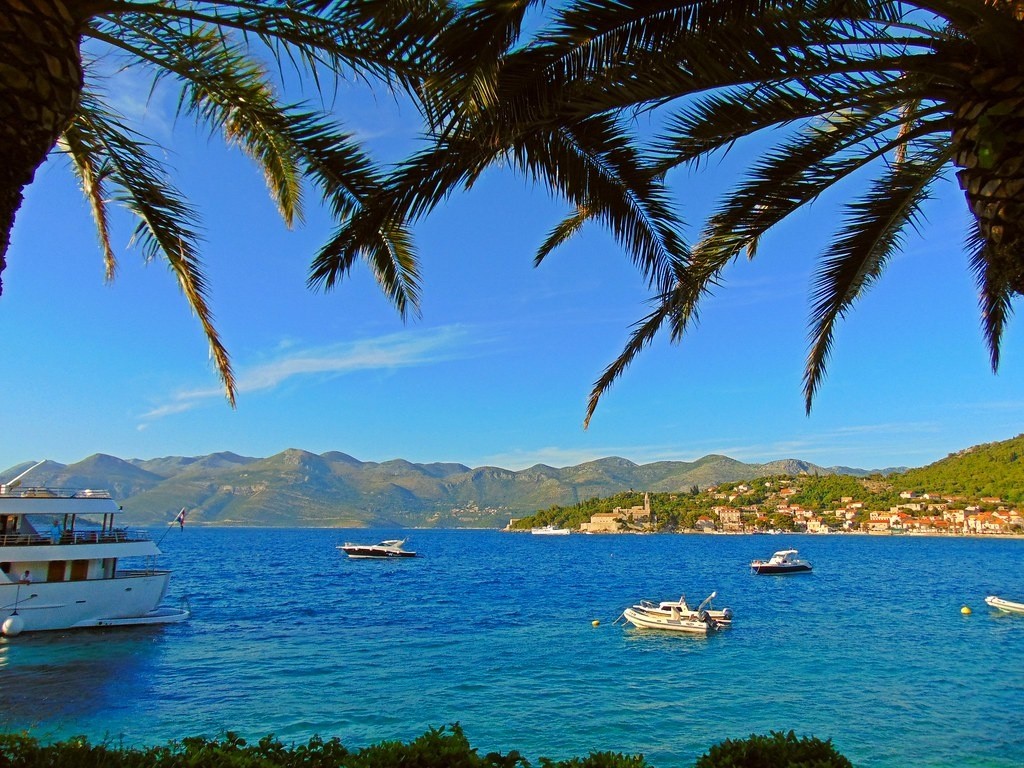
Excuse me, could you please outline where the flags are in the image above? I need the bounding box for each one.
[176,508,185,532]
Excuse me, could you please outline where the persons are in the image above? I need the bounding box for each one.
[19,570,32,585]
[44,520,64,545]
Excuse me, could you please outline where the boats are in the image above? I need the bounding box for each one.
[335,536,419,560]
[0,459,193,637]
[748,548,814,577]
[612,590,732,636]
[985,594,1024,616]
